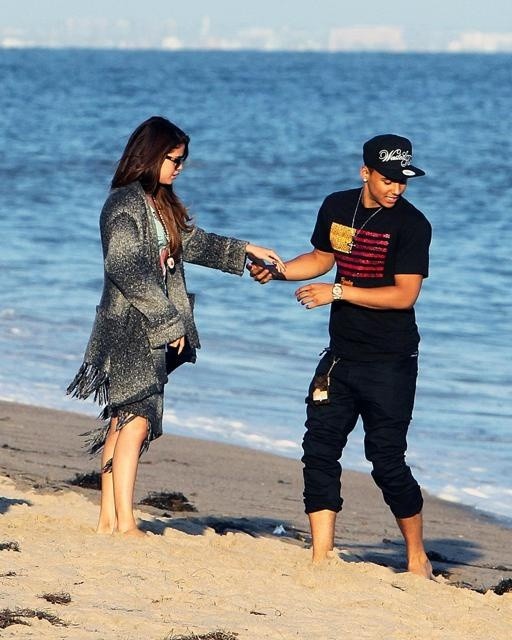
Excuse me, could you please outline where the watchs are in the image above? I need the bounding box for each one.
[332,283,346,303]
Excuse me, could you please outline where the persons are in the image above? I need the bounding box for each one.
[247,136,433,578]
[65,115,287,537]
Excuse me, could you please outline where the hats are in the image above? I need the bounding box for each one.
[363,134,424,180]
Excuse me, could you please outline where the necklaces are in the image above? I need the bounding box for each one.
[346,185,381,254]
[148,190,176,272]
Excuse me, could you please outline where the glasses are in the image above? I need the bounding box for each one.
[166,154,187,164]
